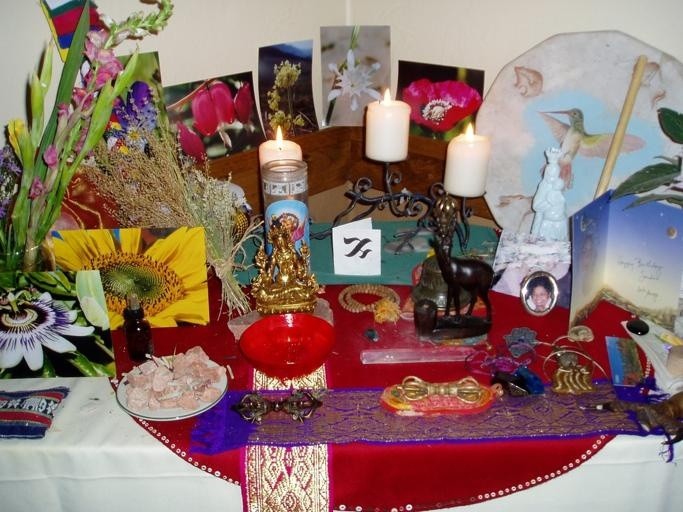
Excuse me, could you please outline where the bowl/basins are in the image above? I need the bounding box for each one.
[240,313,336,378]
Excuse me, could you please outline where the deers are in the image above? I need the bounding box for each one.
[427,238,495,326]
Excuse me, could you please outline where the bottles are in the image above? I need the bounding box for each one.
[122,299,152,363]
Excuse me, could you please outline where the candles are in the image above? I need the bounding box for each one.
[259,125,303,172]
[443,122,492,198]
[365,88,412,162]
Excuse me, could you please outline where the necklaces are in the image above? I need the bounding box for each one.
[338,283,400,316]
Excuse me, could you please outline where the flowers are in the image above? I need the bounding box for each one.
[178,79,253,163]
[266,59,305,138]
[322,25,383,126]
[402,79,481,142]
[0,0,173,277]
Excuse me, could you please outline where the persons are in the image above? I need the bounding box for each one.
[250,215,317,303]
[525,275,554,311]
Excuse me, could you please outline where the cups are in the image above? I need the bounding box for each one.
[263,160,309,281]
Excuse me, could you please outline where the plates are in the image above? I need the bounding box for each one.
[117,355,229,422]
[476,30,681,233]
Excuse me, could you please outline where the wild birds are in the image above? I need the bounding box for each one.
[535,108,646,191]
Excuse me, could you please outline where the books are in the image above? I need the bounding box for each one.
[620,317,683,398]
